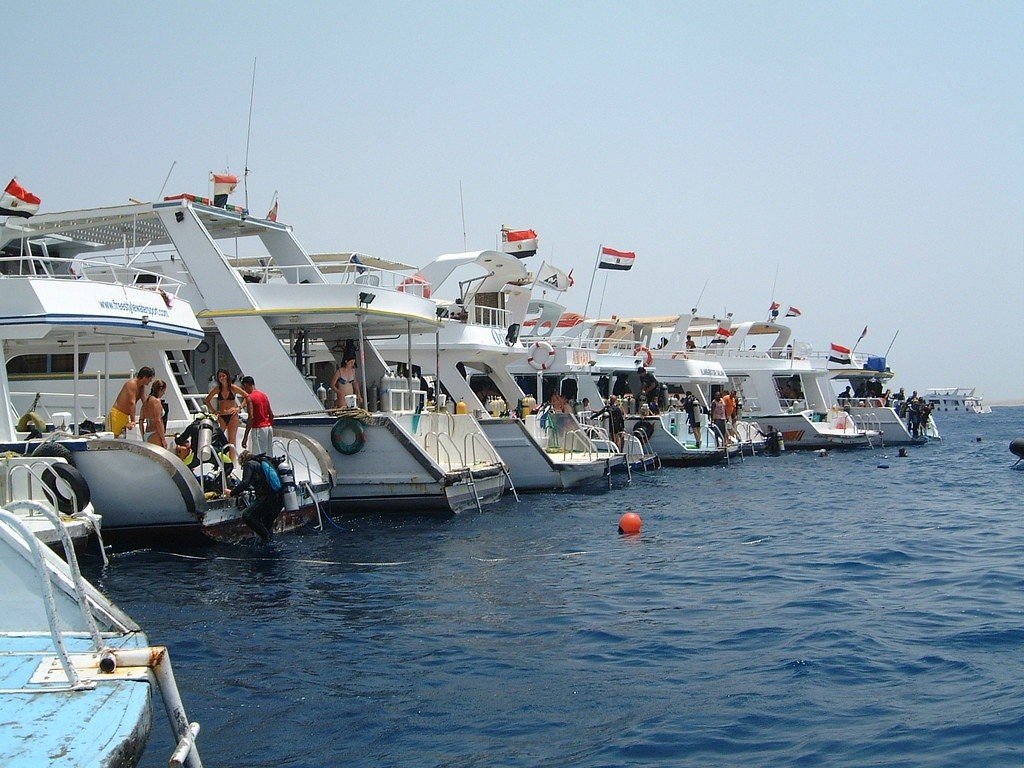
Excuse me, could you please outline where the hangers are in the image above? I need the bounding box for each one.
[332,339,344,352]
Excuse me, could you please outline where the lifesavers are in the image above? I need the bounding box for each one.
[671,352,688,359]
[527,341,555,370]
[634,347,653,367]
[30,443,76,467]
[397,276,430,299]
[632,420,654,439]
[331,417,368,455]
[40,462,92,516]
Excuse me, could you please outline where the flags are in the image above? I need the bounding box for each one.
[351,255,365,274]
[768,302,801,320]
[504,230,538,257]
[269,201,278,223]
[536,263,572,293]
[829,345,850,364]
[0,179,41,218]
[213,174,236,207]
[599,248,635,270]
[710,328,731,344]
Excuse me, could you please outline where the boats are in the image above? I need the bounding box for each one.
[0,56,944,565]
[922,387,992,415]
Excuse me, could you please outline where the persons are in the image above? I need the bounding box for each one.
[331,354,362,407]
[140,380,166,449]
[837,383,933,438]
[637,367,739,449]
[551,389,624,444]
[241,376,273,457]
[660,337,668,348]
[109,367,154,435]
[686,336,695,348]
[224,450,282,543]
[204,369,247,460]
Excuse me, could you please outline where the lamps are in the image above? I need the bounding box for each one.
[588,361,596,366]
[506,324,520,347]
[635,360,642,366]
[359,292,375,308]
[436,308,449,321]
